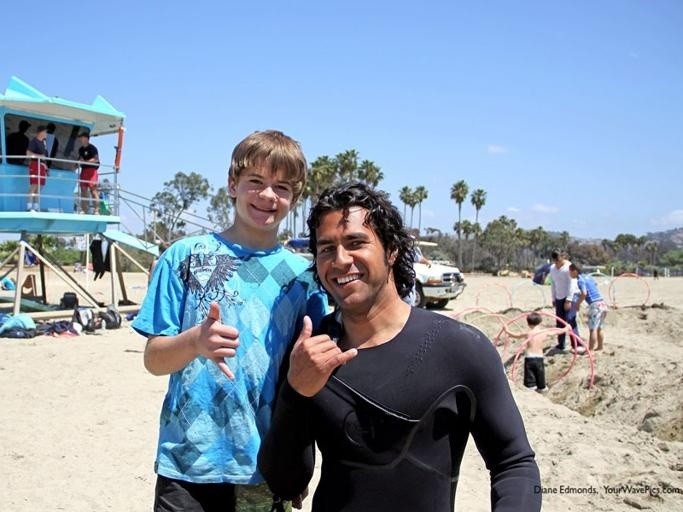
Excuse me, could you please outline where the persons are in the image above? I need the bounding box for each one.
[27,125,49,213]
[415,252,431,268]
[569,262,608,352]
[502,312,570,392]
[130,128,329,512]
[548,248,584,355]
[653,270,658,280]
[77,131,101,215]
[256,179,543,512]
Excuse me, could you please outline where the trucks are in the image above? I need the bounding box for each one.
[403,239,465,309]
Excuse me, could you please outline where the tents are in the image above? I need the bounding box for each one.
[533,264,551,285]
